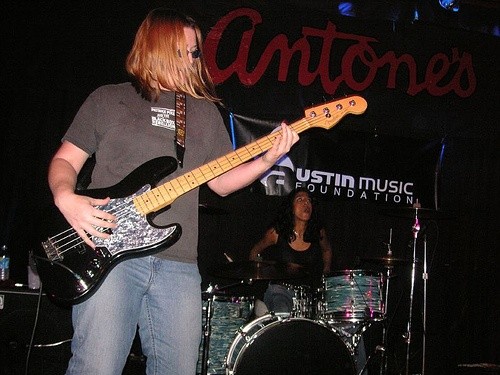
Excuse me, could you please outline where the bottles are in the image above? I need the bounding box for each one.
[0,245,10,280]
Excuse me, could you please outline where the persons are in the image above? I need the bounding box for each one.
[247,188,337,318]
[47,6,300,375]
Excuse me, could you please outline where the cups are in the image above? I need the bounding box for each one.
[28,266,40,289]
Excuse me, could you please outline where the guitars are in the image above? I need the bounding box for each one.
[31,88,369,306]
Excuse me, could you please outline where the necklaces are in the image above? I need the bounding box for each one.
[294,230,303,237]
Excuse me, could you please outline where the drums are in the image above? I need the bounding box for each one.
[196,291,256,375]
[316,266,386,324]
[224,311,359,375]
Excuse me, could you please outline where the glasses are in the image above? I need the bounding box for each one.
[176,48,200,58]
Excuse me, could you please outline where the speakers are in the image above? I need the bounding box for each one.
[0,289,69,375]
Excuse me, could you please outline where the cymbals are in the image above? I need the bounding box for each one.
[376,205,461,221]
[353,253,415,265]
[205,258,310,282]
